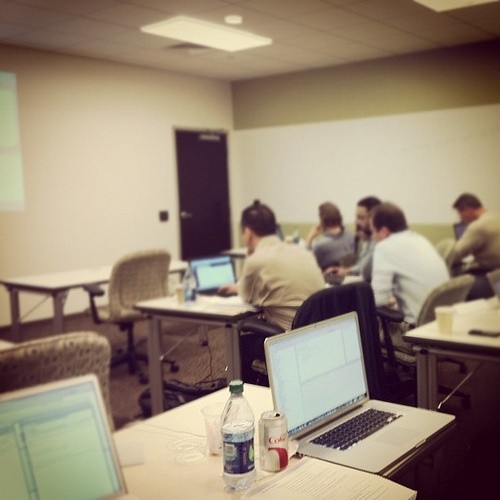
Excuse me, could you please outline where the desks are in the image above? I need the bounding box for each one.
[402,294,500,472]
[0,258,189,341]
[114,381,457,500]
[133,274,362,417]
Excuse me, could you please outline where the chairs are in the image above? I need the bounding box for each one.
[376,273,476,408]
[0,330,113,433]
[82,249,180,384]
[240,281,385,400]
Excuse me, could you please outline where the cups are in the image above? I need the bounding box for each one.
[176,286,185,304]
[201,402,225,457]
[435,306,453,334]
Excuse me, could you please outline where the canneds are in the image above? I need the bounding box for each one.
[258,410,289,472]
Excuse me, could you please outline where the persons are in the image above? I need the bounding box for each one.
[216,203,324,387]
[367,202,451,357]
[306,195,383,278]
[447,193,500,267]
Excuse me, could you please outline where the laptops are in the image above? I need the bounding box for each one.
[189,255,239,297]
[264,311,457,474]
[0,374,130,500]
[487,269,500,300]
[452,223,467,242]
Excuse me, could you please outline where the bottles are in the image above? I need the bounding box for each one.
[183,265,196,304]
[221,380,257,492]
[293,228,300,245]
